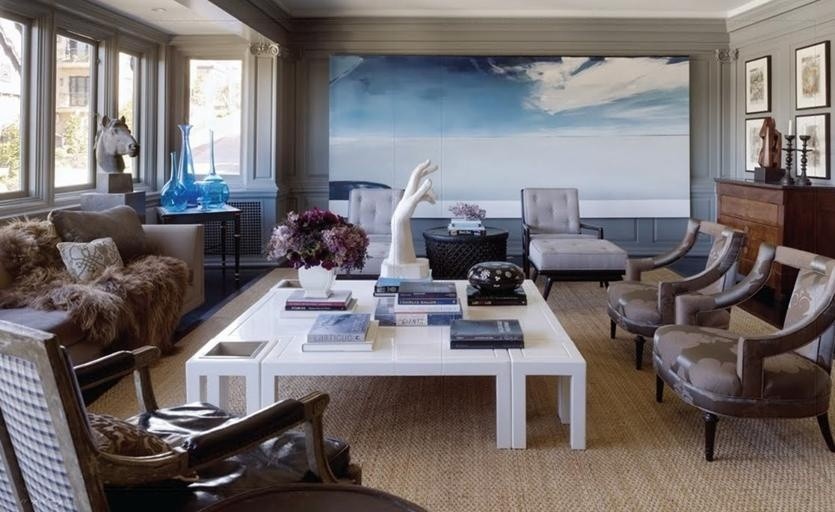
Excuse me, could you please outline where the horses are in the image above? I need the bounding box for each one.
[92,114,141,173]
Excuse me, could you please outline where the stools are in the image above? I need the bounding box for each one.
[530,239,627,301]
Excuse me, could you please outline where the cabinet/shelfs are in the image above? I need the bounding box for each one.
[716,180,835,304]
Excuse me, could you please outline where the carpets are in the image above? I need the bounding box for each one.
[84,266,834,511]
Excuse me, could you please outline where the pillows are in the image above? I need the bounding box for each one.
[87,412,199,482]
[50,206,142,254]
[58,238,124,283]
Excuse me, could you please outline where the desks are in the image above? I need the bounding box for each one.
[159,206,243,288]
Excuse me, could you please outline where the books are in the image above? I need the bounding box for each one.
[307,314,372,343]
[449,319,523,342]
[448,218,487,237]
[450,341,524,350]
[466,284,527,306]
[302,319,379,353]
[373,277,463,327]
[279,290,358,318]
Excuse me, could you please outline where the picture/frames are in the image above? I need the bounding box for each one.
[742,40,831,178]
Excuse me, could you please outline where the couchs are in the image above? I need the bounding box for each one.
[3,222,206,363]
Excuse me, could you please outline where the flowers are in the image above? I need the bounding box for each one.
[266,210,365,271]
[447,204,486,222]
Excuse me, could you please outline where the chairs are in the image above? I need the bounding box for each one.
[0,321,359,512]
[347,188,402,280]
[652,243,834,462]
[519,188,628,300]
[605,219,747,369]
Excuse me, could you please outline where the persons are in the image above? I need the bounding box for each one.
[388,158,438,265]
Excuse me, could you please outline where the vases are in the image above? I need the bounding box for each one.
[160,124,230,211]
[296,268,337,298]
[448,223,487,234]
